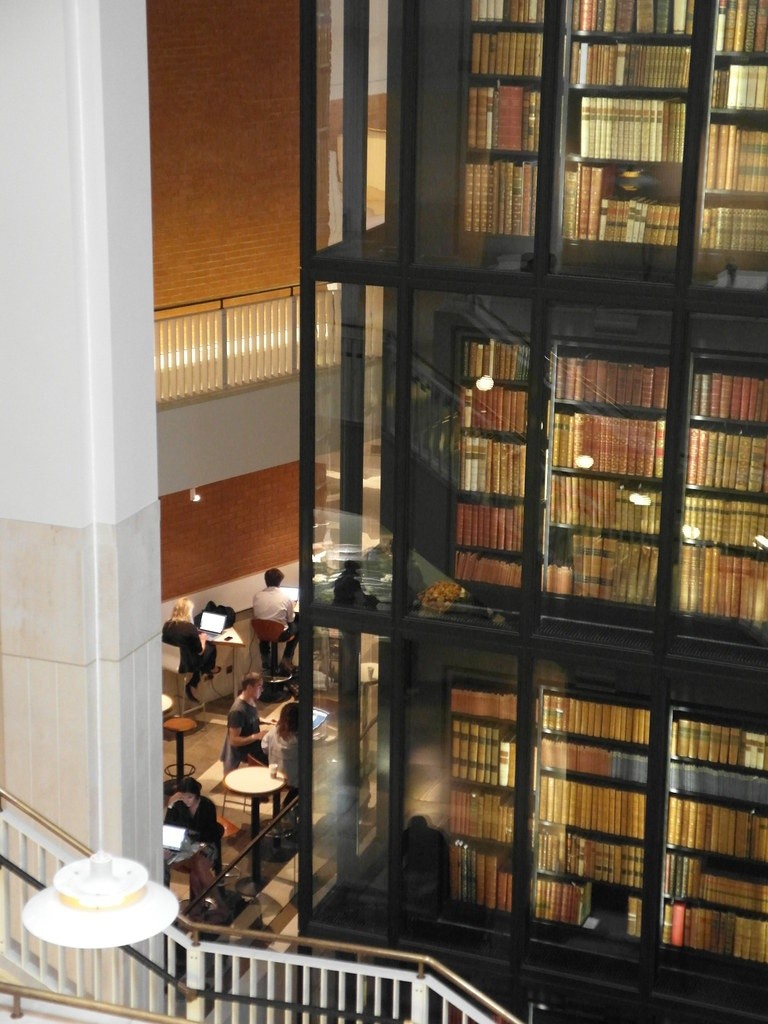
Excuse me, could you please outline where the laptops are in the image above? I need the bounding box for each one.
[196,612,227,641]
[310,707,329,730]
[277,586,298,605]
[162,823,187,866]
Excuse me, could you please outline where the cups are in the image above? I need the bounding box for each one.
[269,763,278,779]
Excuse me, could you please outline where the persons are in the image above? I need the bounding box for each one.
[332,560,379,612]
[161,597,222,704]
[260,702,301,816]
[312,536,394,582]
[251,567,300,674]
[227,671,279,803]
[165,777,231,924]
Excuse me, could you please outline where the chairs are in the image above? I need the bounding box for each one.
[160,531,382,923]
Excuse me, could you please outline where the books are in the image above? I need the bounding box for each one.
[441,1,768,968]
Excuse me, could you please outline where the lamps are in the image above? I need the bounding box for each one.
[18,3,179,950]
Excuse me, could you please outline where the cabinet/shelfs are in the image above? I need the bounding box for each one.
[299,0,768,1022]
